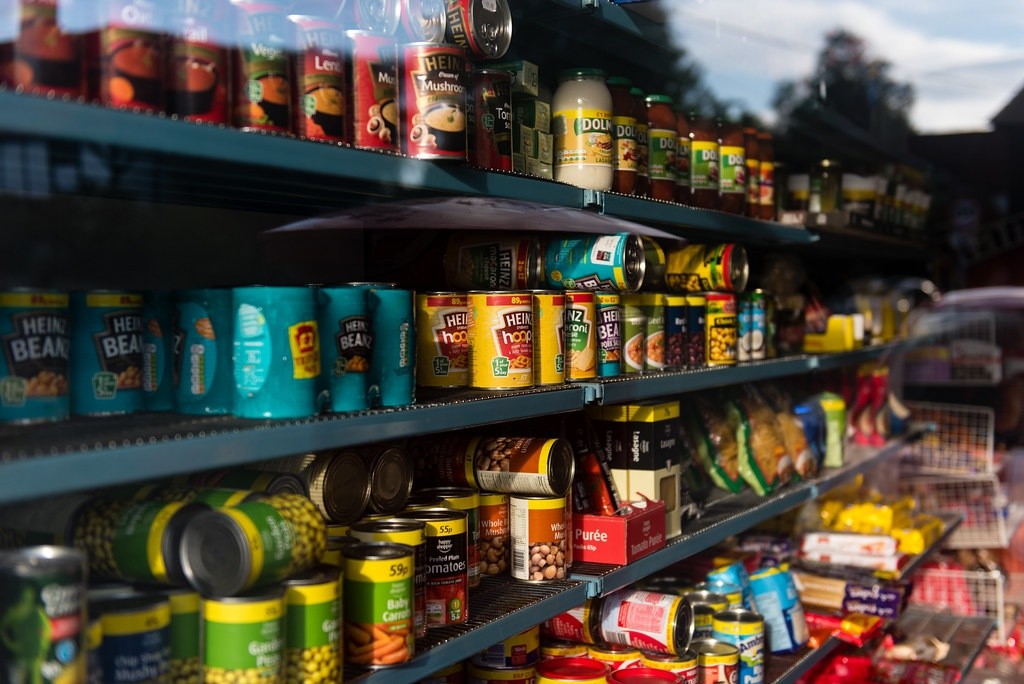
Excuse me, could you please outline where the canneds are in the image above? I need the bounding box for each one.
[0,0,848,684]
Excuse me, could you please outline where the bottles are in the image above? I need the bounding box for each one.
[552,67,614,192]
[644,96,677,203]
[810,159,837,215]
[742,129,760,220]
[674,109,691,205]
[795,389,844,464]
[774,162,785,214]
[689,113,720,210]
[719,125,746,215]
[605,76,650,199]
[756,134,776,220]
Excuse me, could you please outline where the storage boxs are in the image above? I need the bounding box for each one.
[572,400,682,566]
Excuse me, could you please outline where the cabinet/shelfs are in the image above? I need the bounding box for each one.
[0,87,994,684]
